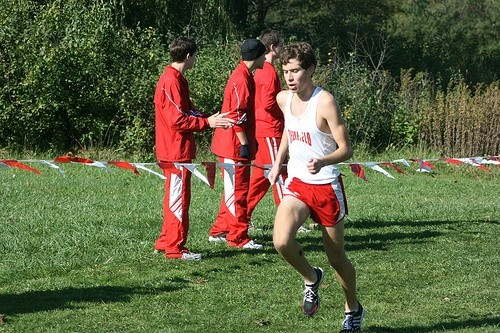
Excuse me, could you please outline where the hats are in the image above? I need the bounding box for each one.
[241,39,266,61]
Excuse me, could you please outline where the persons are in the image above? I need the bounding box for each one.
[268,41,364,333]
[247,33,312,233]
[153,38,236,260]
[209,38,267,250]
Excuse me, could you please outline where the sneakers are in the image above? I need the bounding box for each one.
[340,300,364,333]
[208,233,228,243]
[301,266,323,316]
[236,240,263,250]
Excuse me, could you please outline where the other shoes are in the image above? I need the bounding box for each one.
[296,226,312,233]
[247,221,262,233]
[153,249,165,254]
[180,250,201,261]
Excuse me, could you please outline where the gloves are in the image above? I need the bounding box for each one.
[240,144,251,158]
